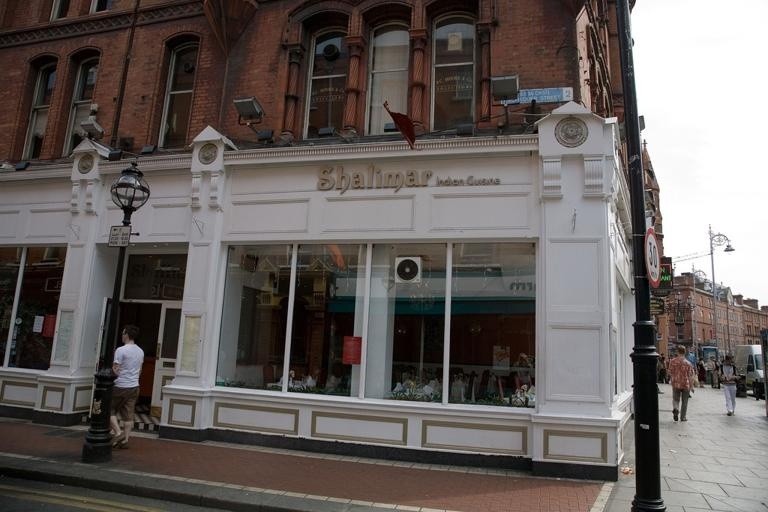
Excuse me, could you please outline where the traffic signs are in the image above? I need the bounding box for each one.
[643,227,662,290]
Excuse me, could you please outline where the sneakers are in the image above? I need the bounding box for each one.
[727,410,735,416]
[681,417,687,421]
[672,408,678,421]
[112,432,128,449]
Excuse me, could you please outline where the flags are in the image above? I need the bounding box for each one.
[391,112,415,145]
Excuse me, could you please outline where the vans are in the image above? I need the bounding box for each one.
[734,344,766,400]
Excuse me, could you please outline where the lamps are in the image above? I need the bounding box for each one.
[233,97,264,135]
[80,119,104,140]
[487,75,519,130]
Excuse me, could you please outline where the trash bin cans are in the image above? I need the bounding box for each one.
[737,373,747,397]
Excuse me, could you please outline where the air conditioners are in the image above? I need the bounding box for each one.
[395,257,424,286]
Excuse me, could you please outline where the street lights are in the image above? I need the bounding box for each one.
[707,224,736,389]
[79,153,152,463]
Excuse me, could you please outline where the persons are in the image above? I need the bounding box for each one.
[718,356,741,416]
[110,325,144,449]
[515,353,532,391]
[659,346,724,398]
[668,345,694,421]
